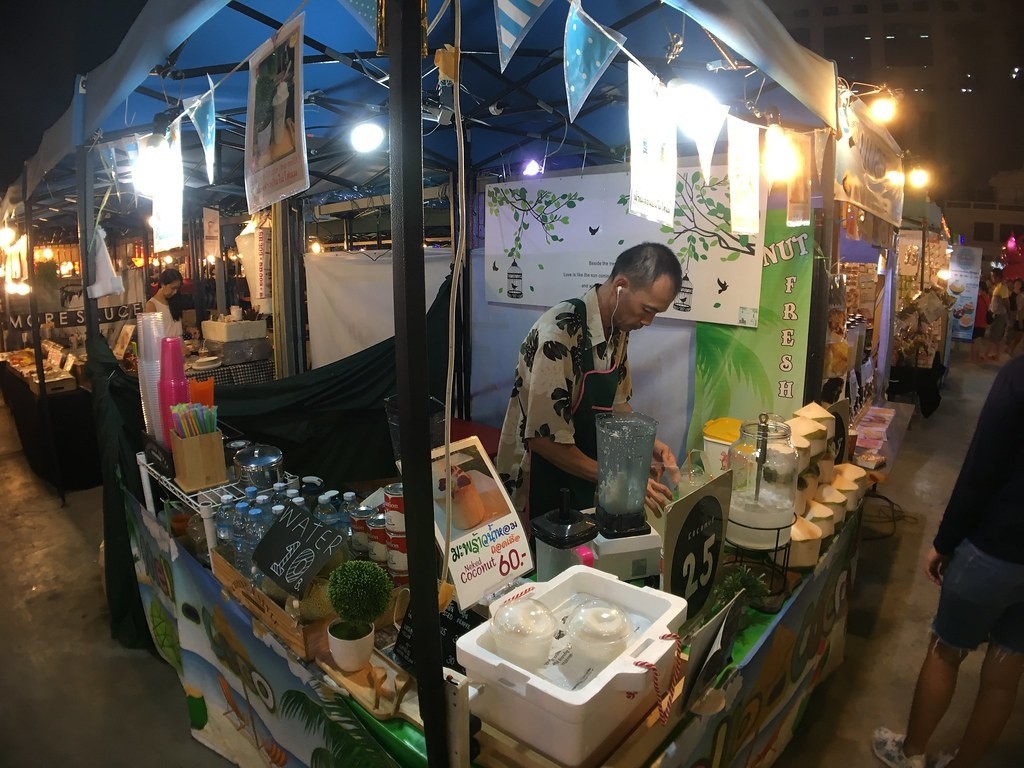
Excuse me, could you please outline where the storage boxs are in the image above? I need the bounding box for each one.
[202,319,273,366]
[456,565,689,768]
[141,418,244,501]
[702,416,744,480]
[6,354,77,395]
[211,544,411,661]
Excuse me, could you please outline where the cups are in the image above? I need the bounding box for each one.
[272,94,289,145]
[531,508,597,582]
[677,462,707,500]
[434,473,486,531]
[135,312,191,450]
[230,308,243,321]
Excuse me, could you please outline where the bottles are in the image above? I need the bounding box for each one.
[216,483,311,587]
[726,417,797,549]
[314,489,342,530]
[301,476,323,510]
[339,491,358,538]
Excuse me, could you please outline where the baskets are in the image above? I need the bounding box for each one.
[141,419,245,478]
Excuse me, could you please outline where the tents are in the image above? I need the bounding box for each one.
[20,0,898,767]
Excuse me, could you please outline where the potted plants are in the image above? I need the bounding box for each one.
[326,559,393,672]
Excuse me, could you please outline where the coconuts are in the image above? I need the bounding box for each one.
[768,401,866,570]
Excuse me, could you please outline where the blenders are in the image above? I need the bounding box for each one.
[578,411,662,590]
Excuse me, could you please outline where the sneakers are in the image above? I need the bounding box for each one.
[929,747,960,768]
[872,726,926,768]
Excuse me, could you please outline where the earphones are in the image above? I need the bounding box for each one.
[617,286,622,296]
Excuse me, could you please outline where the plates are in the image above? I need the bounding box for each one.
[192,363,221,371]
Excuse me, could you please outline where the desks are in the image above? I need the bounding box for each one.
[0,348,275,508]
[848,396,916,536]
[120,497,865,768]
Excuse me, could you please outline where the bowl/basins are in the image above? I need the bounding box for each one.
[565,599,631,671]
[488,598,559,669]
[196,356,218,366]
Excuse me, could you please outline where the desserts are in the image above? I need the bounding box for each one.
[963,303,974,314]
[960,314,972,329]
[432,461,484,529]
[954,308,964,318]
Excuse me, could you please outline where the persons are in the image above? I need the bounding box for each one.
[495,242,682,578]
[869,353,1024,768]
[968,280,990,364]
[979,267,1012,363]
[145,268,185,339]
[1003,277,1024,357]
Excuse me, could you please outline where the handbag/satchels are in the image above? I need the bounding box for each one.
[986,311,1000,325]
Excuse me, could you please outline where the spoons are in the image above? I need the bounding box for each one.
[370,665,387,709]
[390,671,409,715]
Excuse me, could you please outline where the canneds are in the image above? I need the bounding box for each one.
[388,570,409,587]
[386,530,408,572]
[350,507,375,551]
[384,483,405,534]
[366,516,388,563]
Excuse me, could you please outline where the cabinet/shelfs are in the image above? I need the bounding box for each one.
[717,515,793,602]
[135,450,300,574]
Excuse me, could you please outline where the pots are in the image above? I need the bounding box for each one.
[234,443,284,489]
